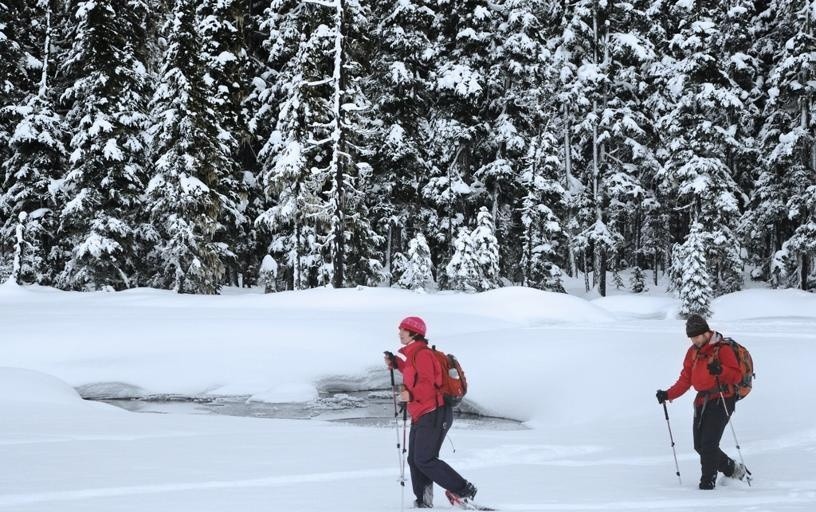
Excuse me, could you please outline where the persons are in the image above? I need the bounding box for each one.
[383,316,479,510]
[655,314,753,490]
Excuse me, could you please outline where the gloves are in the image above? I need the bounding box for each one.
[706,360,723,376]
[656,391,668,404]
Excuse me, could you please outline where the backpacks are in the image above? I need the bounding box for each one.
[711,336,755,400]
[411,344,468,407]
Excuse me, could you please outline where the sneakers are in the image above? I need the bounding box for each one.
[730,463,746,479]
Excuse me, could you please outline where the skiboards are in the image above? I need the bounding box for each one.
[400,490,500,511]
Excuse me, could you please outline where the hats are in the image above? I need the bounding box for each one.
[399,316,426,337]
[686,314,709,338]
